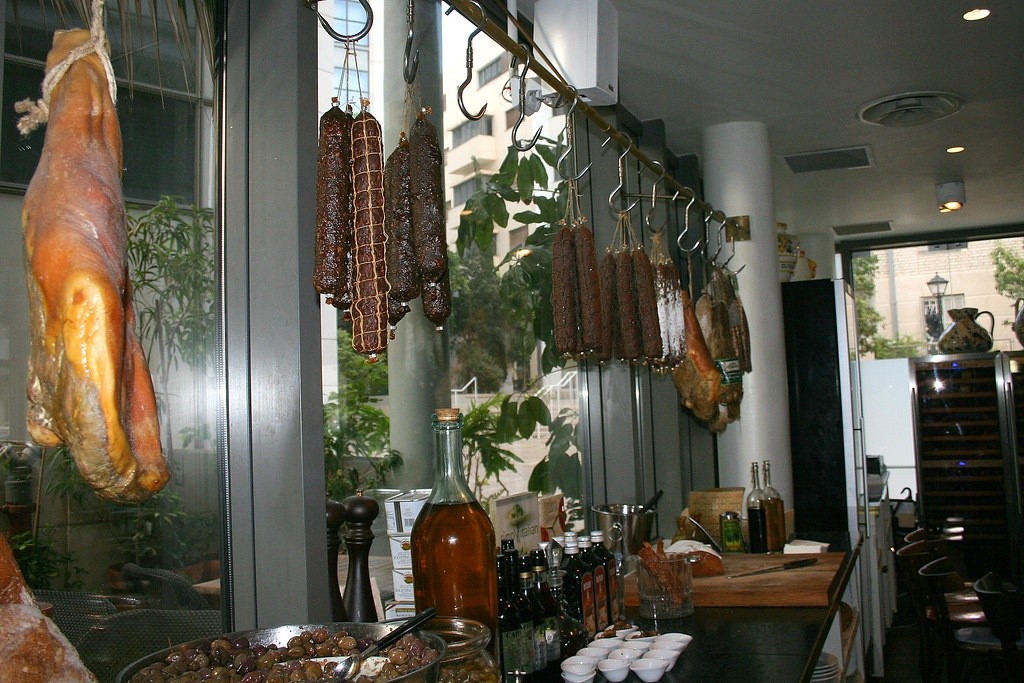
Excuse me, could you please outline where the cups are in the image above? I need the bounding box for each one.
[636,552,694,617]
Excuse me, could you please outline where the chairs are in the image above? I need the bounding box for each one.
[894,524,1024,682]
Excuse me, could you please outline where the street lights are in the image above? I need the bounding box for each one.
[925,272,949,330]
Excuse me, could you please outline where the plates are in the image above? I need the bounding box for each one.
[840,601,853,631]
[810,651,842,682]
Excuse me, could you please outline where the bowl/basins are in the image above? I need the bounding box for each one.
[560,624,694,683]
[113,621,446,683]
[590,503,657,557]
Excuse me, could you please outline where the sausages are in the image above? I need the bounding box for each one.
[312,96,456,363]
[552,219,687,374]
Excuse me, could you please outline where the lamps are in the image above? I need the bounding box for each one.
[936,181,967,213]
[960,8,992,21]
[944,145,965,155]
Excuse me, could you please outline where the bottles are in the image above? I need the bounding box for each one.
[748,459,780,554]
[409,408,625,676]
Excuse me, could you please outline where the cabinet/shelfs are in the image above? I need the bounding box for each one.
[907,352,1023,573]
